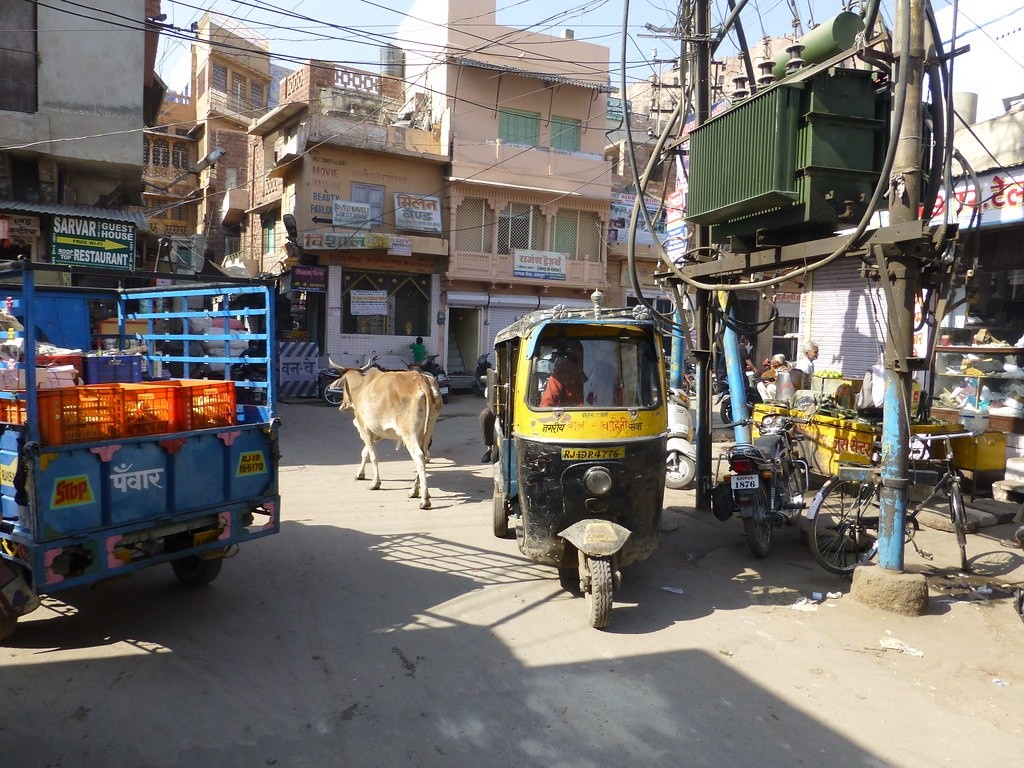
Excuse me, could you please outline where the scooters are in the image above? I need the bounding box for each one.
[666,385,697,488]
[401,352,454,405]
[475,352,495,398]
[663,348,697,396]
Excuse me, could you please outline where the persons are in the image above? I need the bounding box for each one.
[410,336,425,364]
[479,404,498,463]
[738,334,759,387]
[795,341,819,375]
[231,340,267,390]
[539,339,624,407]
[756,353,789,402]
[161,326,206,379]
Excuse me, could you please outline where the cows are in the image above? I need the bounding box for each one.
[328,356,445,510]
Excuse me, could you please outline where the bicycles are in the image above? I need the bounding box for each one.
[810,428,973,577]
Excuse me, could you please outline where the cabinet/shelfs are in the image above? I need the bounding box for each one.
[924,345,1024,432]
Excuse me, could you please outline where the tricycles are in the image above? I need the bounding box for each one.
[0,254,282,645]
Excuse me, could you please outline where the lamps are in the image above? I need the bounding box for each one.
[142,147,226,191]
[283,214,305,261]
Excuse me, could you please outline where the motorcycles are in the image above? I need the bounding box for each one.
[726,410,813,560]
[314,350,386,408]
[485,288,670,629]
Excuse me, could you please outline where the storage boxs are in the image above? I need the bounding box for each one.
[0,351,236,447]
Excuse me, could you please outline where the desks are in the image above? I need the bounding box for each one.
[751,402,1006,503]
